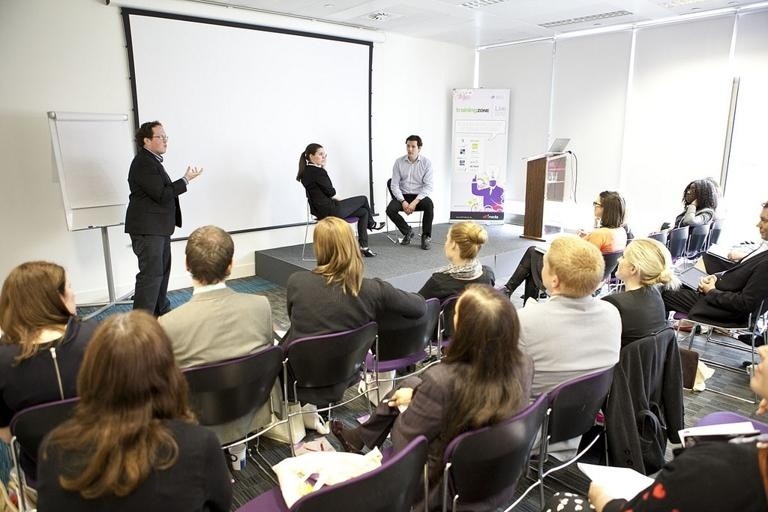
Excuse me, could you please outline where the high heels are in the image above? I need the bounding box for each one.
[367,221,387,232]
[400,228,415,245]
[421,232,433,251]
[359,246,378,258]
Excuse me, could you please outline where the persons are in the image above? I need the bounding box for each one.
[1,190,768,512]
[297,143,385,258]
[126,121,205,315]
[660,177,717,230]
[385,135,435,250]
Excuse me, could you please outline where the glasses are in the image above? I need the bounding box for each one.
[148,133,170,142]
[593,200,603,208]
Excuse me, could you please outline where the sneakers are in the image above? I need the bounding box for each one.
[328,416,363,455]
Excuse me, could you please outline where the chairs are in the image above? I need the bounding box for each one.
[382,177,434,243]
[303,186,366,276]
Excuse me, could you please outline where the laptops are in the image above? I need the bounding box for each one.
[546,138,570,156]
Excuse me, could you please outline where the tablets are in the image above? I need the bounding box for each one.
[676,266,713,291]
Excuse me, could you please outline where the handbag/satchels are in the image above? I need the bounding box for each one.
[676,347,699,391]
[249,398,309,446]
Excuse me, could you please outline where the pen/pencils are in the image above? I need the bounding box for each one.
[382,398,397,403]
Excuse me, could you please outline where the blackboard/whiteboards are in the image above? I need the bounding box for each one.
[50,113,138,232]
